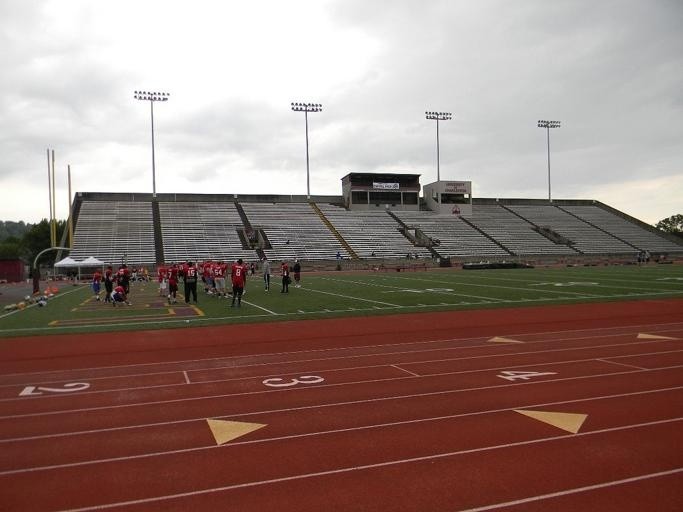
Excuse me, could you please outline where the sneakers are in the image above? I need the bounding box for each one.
[203,289,246,307]
[295,285,301,289]
[168,295,178,303]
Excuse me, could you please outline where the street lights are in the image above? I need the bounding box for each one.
[536,118,563,205]
[424,110,453,183]
[132,88,170,199]
[290,100,323,202]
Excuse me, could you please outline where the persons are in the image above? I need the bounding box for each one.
[637,246,668,267]
[90,257,301,308]
